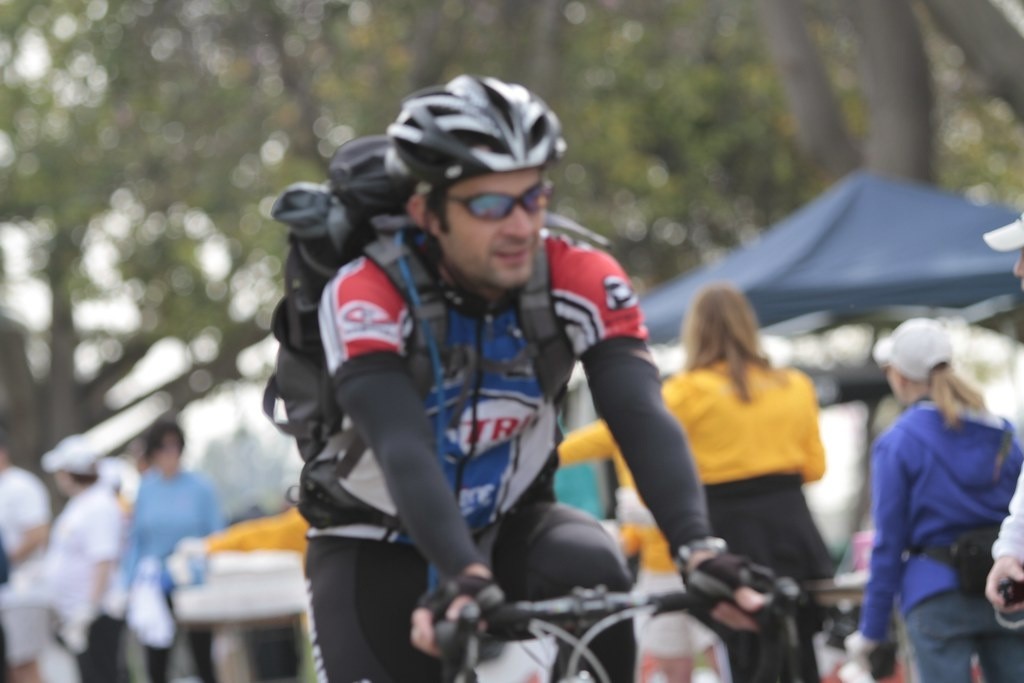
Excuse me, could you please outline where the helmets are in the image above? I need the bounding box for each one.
[383,73,566,203]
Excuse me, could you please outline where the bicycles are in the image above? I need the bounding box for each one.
[434,576,763,683]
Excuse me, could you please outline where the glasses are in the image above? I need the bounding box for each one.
[439,178,554,220]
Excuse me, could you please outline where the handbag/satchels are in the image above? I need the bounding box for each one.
[914,525,998,599]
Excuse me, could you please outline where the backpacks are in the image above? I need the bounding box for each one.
[261,131,575,533]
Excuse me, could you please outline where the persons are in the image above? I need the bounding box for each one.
[555,283,831,622]
[844,316,1024,682]
[982,206,1024,632]
[295,76,813,683]
[0,418,227,683]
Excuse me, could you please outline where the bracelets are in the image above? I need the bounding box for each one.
[674,536,728,573]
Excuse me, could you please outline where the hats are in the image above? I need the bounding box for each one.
[40,434,108,477]
[873,318,956,383]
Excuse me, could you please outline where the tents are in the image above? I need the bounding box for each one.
[566,163,1022,396]
